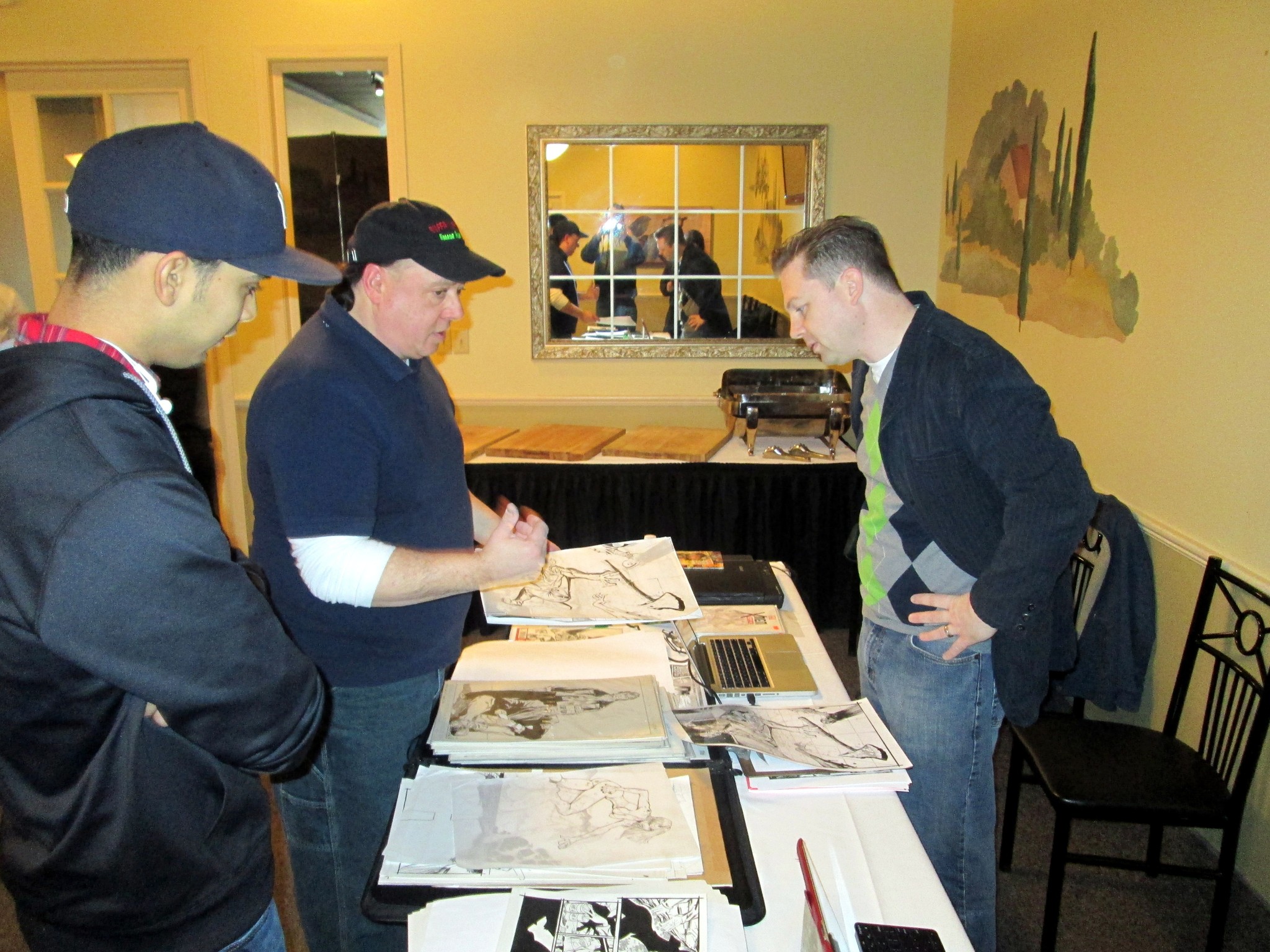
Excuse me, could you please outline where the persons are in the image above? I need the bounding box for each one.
[548,214,600,340]
[654,223,734,339]
[247,198,550,952]
[580,205,646,333]
[0,121,350,952]
[770,213,1099,952]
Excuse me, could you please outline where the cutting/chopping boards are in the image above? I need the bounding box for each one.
[458,424,519,462]
[485,424,626,462]
[602,425,733,463]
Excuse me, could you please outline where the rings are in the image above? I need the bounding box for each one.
[944,624,954,638]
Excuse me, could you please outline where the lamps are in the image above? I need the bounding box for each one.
[371,73,385,97]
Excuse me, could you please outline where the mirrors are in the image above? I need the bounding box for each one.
[526,123,828,359]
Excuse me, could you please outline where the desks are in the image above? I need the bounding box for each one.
[468,437,868,633]
[508,560,976,952]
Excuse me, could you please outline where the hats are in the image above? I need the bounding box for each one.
[347,197,506,283]
[65,121,343,287]
[553,220,590,238]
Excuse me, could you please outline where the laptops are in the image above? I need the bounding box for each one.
[670,616,820,696]
[795,839,949,952]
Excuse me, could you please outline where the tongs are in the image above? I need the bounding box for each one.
[762,445,810,462]
[789,443,831,460]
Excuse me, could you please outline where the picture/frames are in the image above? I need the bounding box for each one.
[623,206,712,267]
[781,144,807,205]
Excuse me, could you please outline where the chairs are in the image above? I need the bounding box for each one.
[1045,495,1158,718]
[1000,557,1270,952]
[732,295,778,338]
